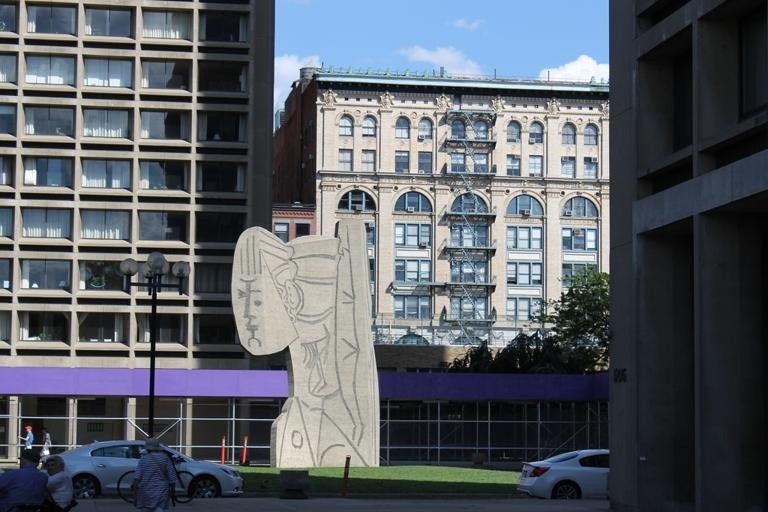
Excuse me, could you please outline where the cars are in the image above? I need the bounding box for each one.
[517,449,610,499]
[41,439,246,498]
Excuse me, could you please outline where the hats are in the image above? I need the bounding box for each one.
[18,449,41,461]
[141,438,165,450]
[25,426,32,429]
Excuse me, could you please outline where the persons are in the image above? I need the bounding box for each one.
[130,437,178,511]
[17,425,34,449]
[37,426,52,470]
[45,455,74,511]
[0,448,49,511]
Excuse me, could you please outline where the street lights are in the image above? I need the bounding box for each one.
[119,252,191,438]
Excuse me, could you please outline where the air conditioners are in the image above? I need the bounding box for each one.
[523,138,598,216]
[355,134,426,333]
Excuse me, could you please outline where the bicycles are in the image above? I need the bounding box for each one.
[117,458,198,503]
